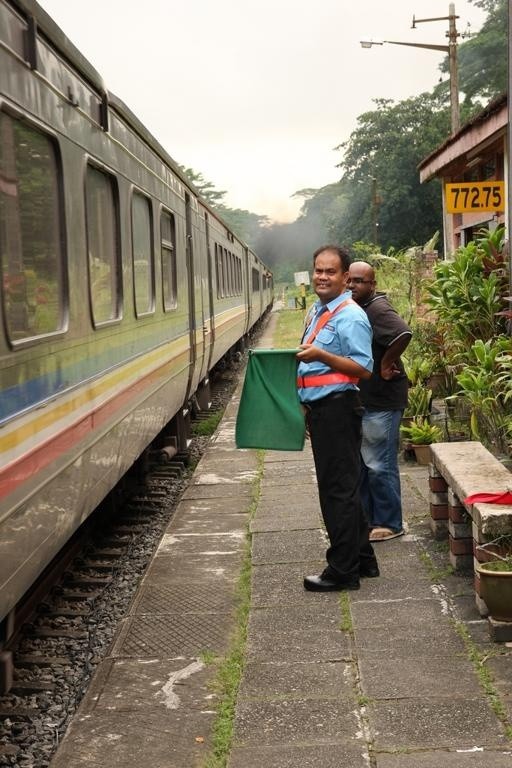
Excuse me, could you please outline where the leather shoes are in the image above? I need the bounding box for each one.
[358,565,379,577]
[304,566,360,592]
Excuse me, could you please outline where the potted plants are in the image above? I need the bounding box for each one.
[399,417,443,465]
[476,557,512,624]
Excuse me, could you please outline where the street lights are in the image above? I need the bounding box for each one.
[360,37,457,135]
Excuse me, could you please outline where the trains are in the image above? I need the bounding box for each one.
[2,0,274,690]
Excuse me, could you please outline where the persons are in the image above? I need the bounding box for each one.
[296,245,381,592]
[342,260,413,540]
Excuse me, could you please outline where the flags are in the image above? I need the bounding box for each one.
[235,347,308,451]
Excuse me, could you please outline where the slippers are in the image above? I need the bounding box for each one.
[368,525,406,541]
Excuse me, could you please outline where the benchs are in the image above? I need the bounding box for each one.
[424,441,512,617]
